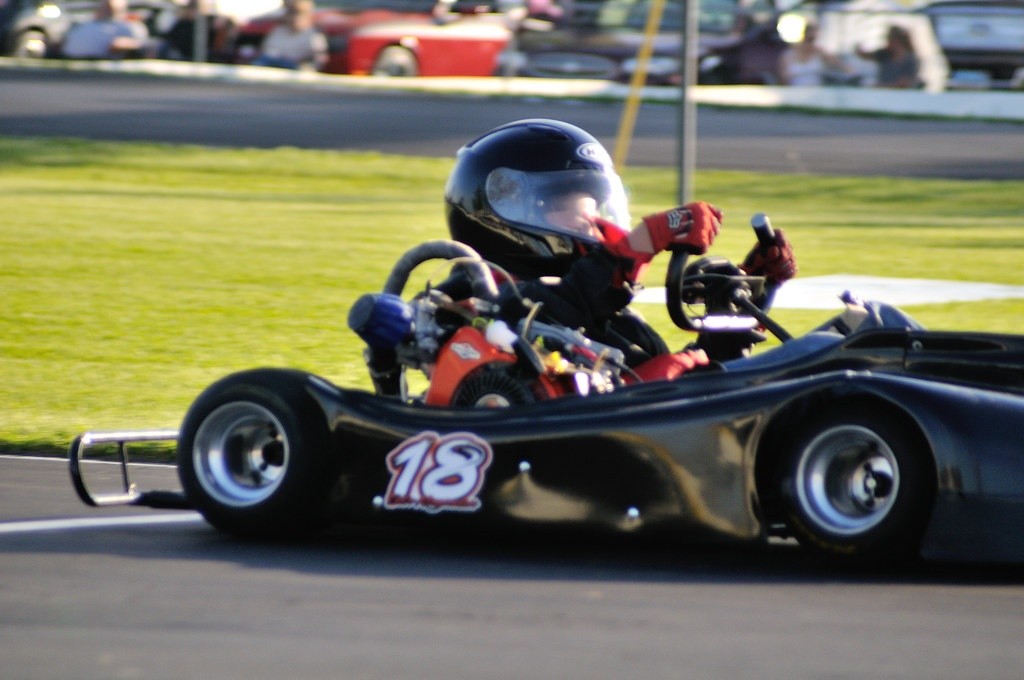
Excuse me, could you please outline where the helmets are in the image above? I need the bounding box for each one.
[444,118,617,279]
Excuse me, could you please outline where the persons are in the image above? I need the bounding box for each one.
[407,118,925,409]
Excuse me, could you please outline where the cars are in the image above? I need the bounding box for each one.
[231,2,514,76]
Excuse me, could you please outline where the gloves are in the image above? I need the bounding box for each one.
[739,231,797,286]
[644,200,724,256]
